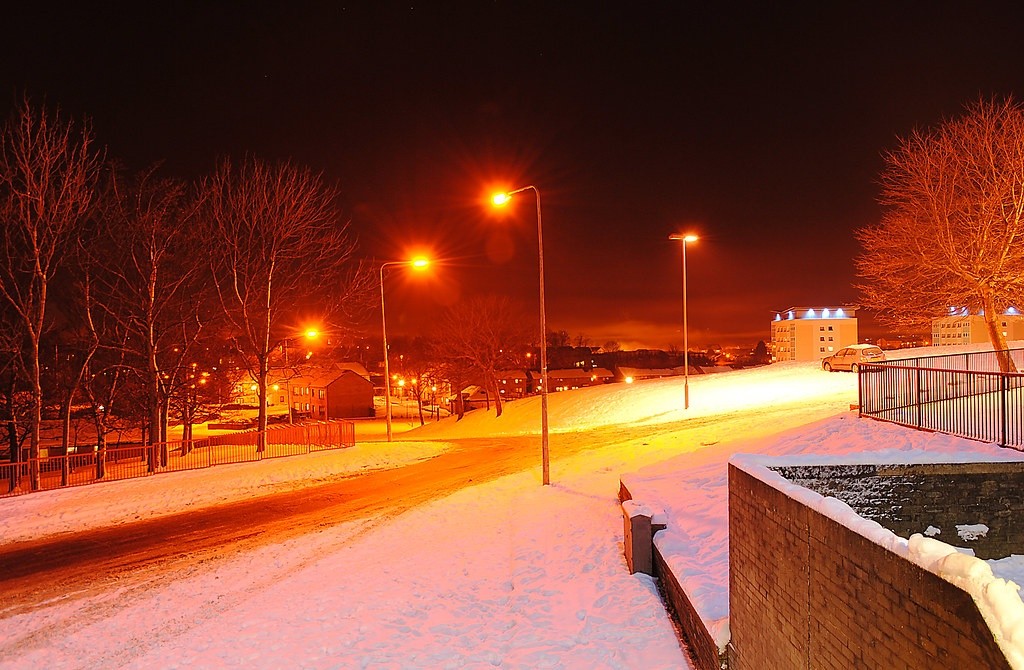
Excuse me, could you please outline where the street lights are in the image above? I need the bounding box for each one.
[670,232,698,409]
[380,259,428,441]
[495,186,548,484]
[286,330,317,424]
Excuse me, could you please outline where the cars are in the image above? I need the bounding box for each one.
[822,344,887,373]
